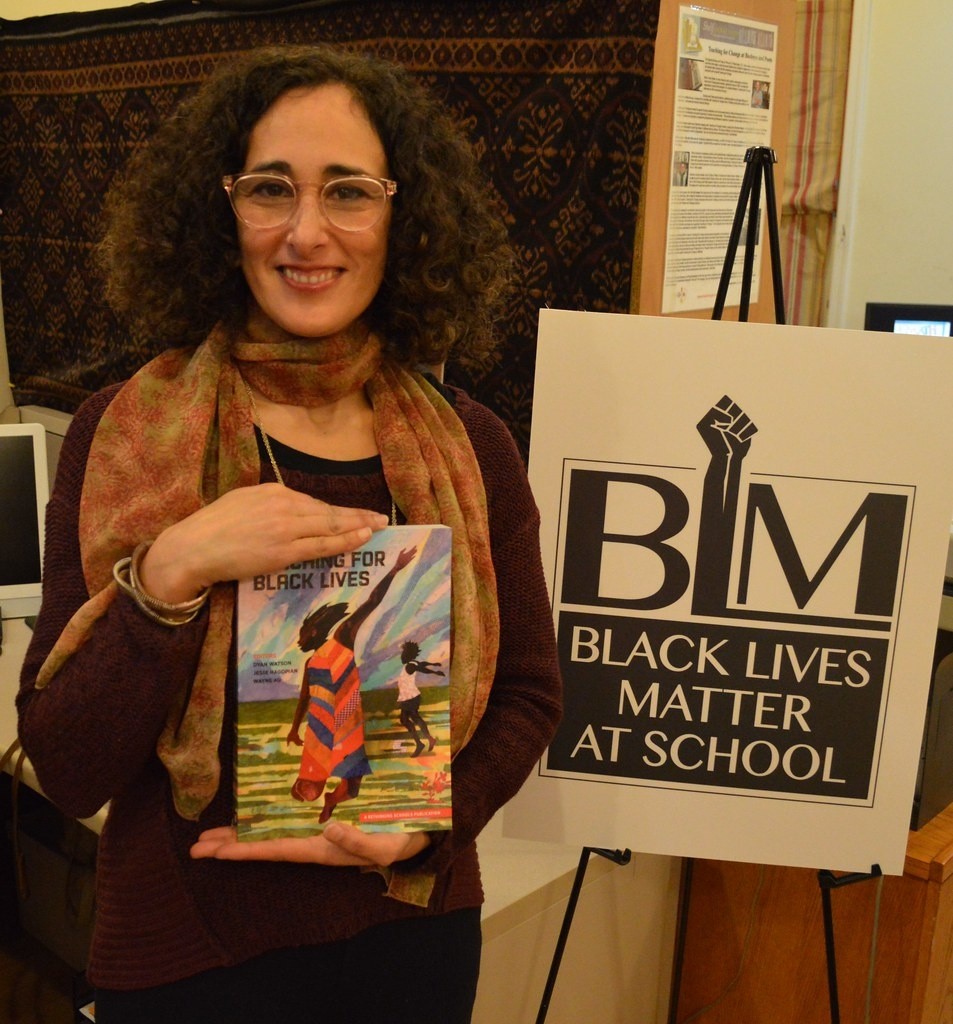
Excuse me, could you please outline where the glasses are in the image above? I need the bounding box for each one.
[221,173,397,233]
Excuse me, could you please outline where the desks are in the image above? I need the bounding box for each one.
[0,614,684,1024]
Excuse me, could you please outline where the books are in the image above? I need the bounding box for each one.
[236,523,453,840]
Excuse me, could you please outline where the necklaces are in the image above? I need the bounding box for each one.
[243,379,396,525]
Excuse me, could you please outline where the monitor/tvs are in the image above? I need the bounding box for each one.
[864,302,953,337]
[0,422,49,600]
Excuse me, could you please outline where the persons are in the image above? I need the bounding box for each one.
[752,82,763,108]
[16,54,562,1024]
[673,163,688,186]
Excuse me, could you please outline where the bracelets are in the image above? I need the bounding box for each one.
[112,540,209,624]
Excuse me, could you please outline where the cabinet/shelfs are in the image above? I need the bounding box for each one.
[676,802,952,1024]
